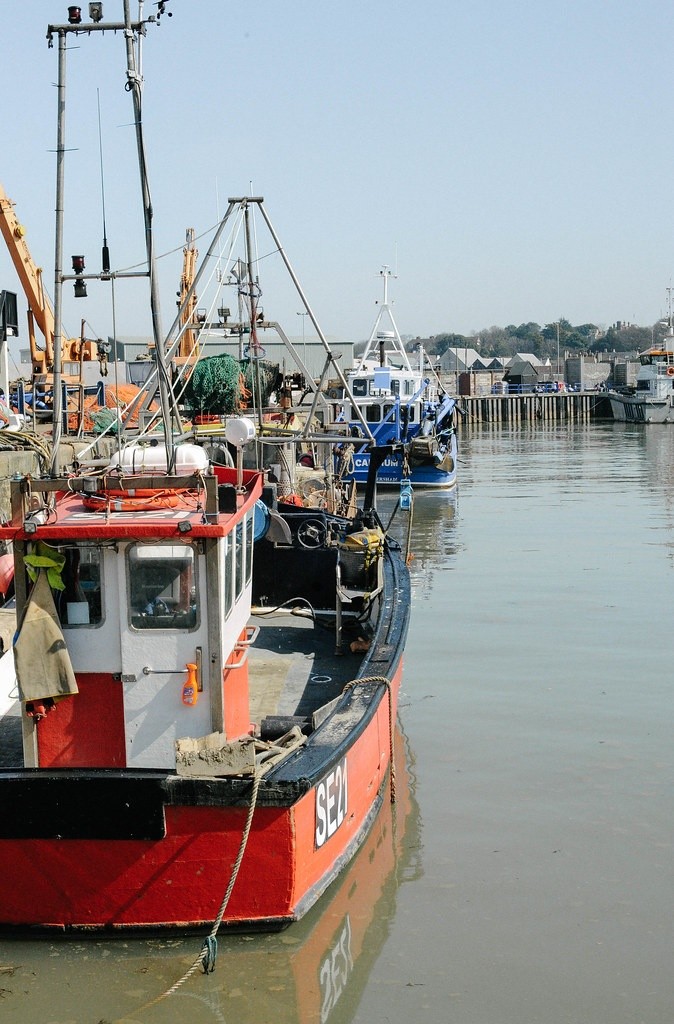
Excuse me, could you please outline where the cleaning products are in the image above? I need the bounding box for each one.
[182,664,197,706]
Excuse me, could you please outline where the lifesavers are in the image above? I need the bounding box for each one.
[83,488,193,512]
[667,367,674,376]
[297,454,314,467]
[194,415,220,424]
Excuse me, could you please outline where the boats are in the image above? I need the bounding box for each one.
[608,388,673,424]
[0,1,461,943]
